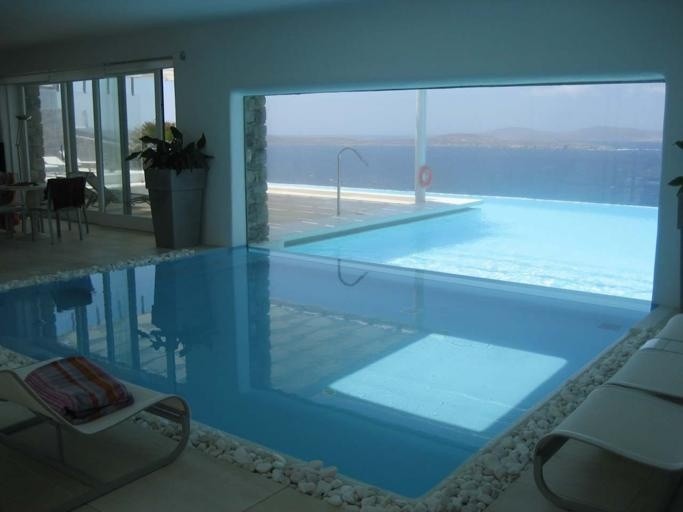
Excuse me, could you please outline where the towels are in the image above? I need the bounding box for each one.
[23,357,135,428]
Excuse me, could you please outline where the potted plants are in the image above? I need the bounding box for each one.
[127,126,213,250]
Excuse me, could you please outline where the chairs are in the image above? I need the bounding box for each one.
[0,168,92,243]
[532,312,683,512]
[0,356,191,512]
